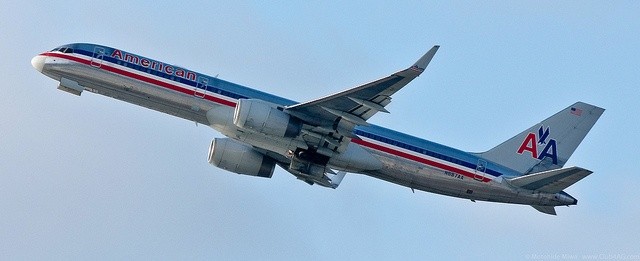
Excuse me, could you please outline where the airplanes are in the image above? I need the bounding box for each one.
[31,43,605,215]
